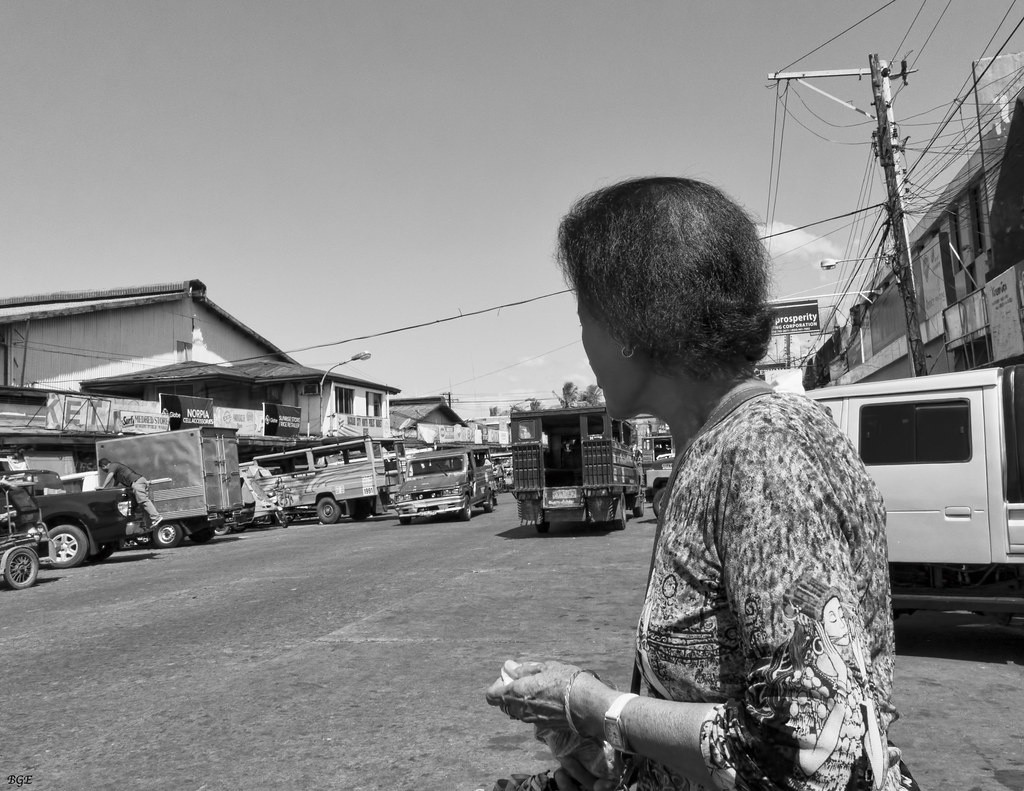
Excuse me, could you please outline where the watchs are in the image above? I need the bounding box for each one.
[603,692,639,756]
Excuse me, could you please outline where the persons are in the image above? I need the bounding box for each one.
[493,458,505,486]
[485,178,914,791]
[96,458,163,529]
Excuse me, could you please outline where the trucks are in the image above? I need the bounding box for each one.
[59,423,244,548]
[213,404,675,536]
[795,362,1024,625]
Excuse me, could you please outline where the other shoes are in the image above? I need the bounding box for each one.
[150,516,164,530]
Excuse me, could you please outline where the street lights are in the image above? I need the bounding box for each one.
[319,351,373,439]
[820,253,929,377]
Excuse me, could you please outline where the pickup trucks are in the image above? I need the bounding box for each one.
[0,468,135,569]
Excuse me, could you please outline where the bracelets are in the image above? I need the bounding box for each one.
[565,669,601,736]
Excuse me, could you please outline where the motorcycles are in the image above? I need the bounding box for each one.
[0,474,56,590]
